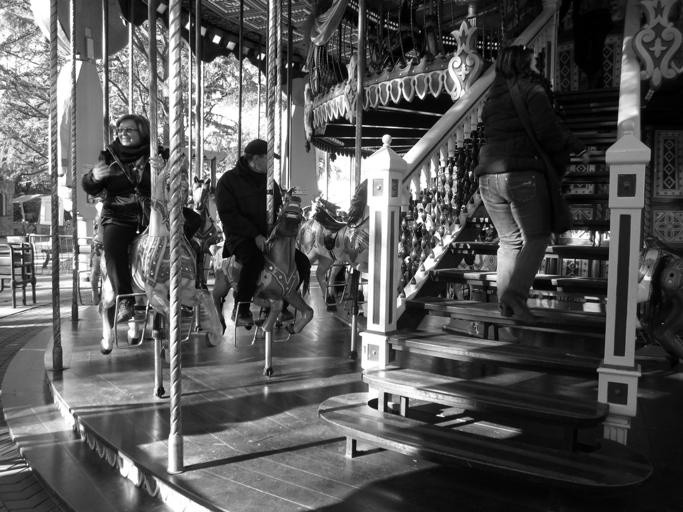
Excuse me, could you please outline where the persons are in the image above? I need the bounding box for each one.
[215,139,310,326]
[80,114,199,323]
[472,44,580,325]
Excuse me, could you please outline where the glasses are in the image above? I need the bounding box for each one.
[115,126,141,134]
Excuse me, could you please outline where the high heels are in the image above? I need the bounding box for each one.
[498,290,539,322]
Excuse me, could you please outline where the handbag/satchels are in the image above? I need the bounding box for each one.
[543,157,571,235]
[139,197,203,242]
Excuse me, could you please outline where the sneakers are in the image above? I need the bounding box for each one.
[231,308,254,327]
[115,298,136,324]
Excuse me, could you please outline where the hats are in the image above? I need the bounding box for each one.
[244,138,281,159]
